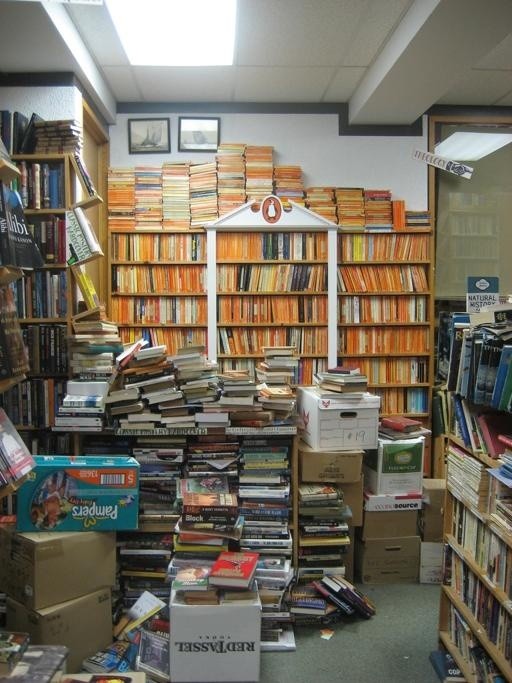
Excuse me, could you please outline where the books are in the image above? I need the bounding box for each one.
[2,111,103,317]
[109,143,430,230]
[110,234,207,358]
[432,312,510,681]
[216,231,328,386]
[336,233,430,426]
[1,313,432,680]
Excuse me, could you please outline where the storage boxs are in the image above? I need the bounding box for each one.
[421,479,446,542]
[337,472,364,526]
[357,511,418,541]
[0,523,116,609]
[355,536,421,585]
[296,386,381,452]
[420,542,442,584]
[16,455,140,531]
[169,579,262,682]
[298,439,366,483]
[363,436,425,495]
[5,587,114,672]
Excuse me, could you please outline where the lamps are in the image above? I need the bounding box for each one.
[434,126,512,161]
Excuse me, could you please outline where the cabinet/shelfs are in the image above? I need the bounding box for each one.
[0,142,100,501]
[437,385,512,683]
[107,196,435,479]
[49,431,300,588]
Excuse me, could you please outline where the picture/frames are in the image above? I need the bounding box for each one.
[177,116,220,152]
[128,118,171,153]
